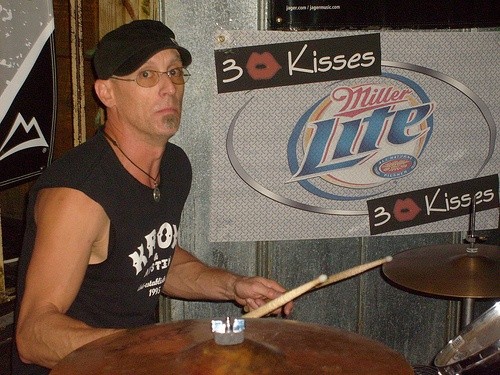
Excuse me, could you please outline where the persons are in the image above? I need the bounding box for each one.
[10,19,294,375]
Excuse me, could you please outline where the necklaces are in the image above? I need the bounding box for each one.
[101,129,162,203]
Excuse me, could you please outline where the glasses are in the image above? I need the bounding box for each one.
[110,69,192,85]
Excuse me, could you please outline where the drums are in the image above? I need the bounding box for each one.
[434,300,500,375]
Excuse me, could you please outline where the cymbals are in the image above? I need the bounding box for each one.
[381,243,500,296]
[49,316,415,375]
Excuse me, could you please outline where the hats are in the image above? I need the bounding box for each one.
[94,18,193,80]
